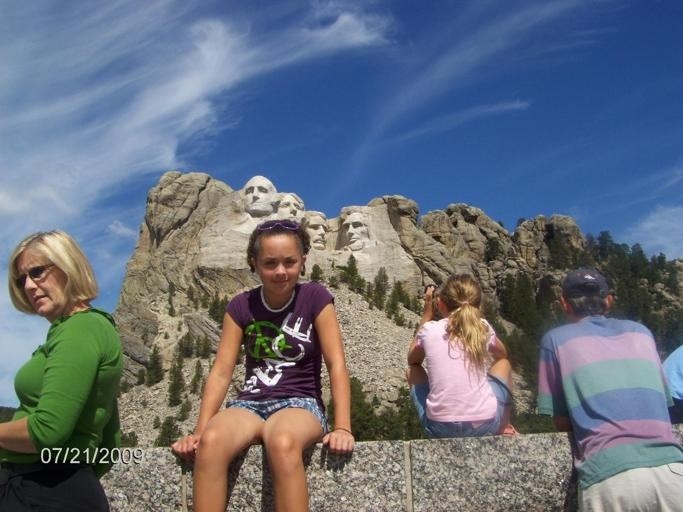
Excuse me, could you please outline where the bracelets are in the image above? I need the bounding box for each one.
[332,427,353,435]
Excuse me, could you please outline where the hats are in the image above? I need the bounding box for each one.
[560,267,608,300]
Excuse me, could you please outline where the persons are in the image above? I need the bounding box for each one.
[534,265,682,512]
[294,210,332,251]
[405,271,523,440]
[0,228,124,511]
[661,340,683,427]
[168,219,356,511]
[227,174,278,235]
[268,190,307,227]
[336,203,373,252]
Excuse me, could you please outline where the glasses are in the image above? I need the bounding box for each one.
[258,218,301,231]
[15,263,55,288]
